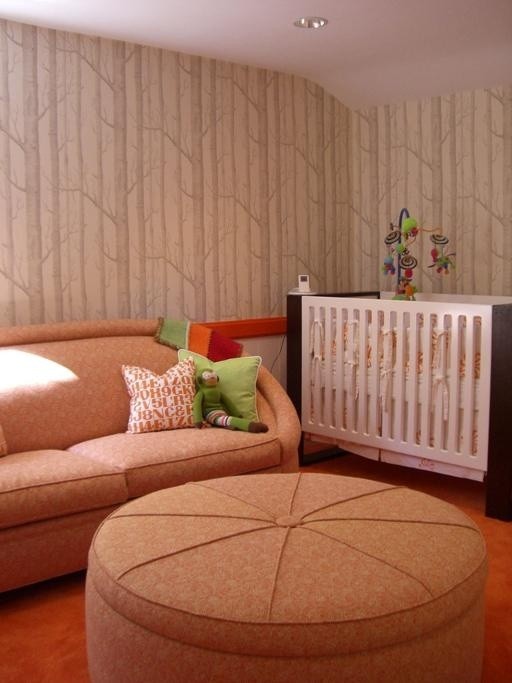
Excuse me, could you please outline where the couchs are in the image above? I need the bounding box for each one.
[80,471,487,678]
[0,318,307,598]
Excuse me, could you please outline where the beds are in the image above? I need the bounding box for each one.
[281,290,512,523]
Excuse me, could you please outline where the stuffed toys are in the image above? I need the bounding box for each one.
[192,368,269,433]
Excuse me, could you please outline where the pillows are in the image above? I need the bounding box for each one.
[117,358,197,436]
[177,346,262,424]
